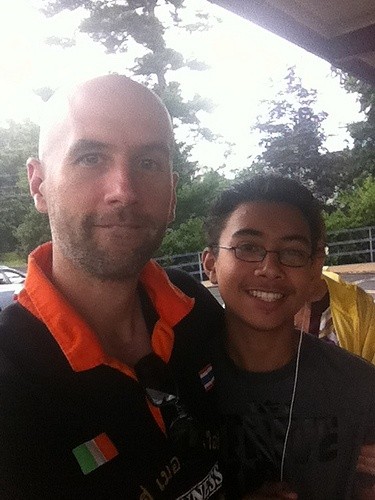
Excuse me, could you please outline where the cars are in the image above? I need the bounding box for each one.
[0,265,29,312]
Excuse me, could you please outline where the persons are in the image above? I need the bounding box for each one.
[293,221,375,367]
[202,173,375,500]
[0,74,243,500]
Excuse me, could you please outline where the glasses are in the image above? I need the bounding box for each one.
[216,241,315,267]
[133,353,205,472]
